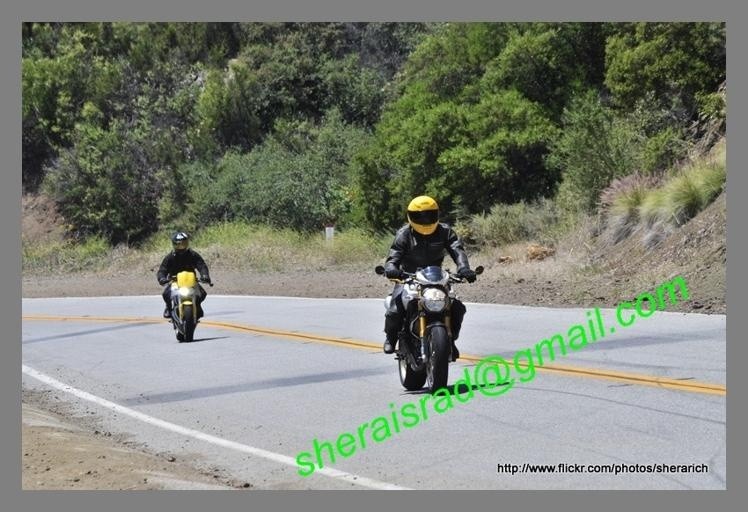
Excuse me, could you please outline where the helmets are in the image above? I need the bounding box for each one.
[171,232,190,254]
[407,195,439,235]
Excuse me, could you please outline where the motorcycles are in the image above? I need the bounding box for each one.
[167,271,210,342]
[375,266,483,395]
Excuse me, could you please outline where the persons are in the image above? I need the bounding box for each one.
[156,231,211,318]
[382,195,477,360]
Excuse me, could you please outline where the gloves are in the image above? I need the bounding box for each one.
[383,262,404,279]
[200,273,210,283]
[157,275,168,286]
[458,266,480,285]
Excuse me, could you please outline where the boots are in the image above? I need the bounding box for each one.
[380,316,399,355]
[162,294,173,319]
[195,291,207,318]
[447,300,469,359]
